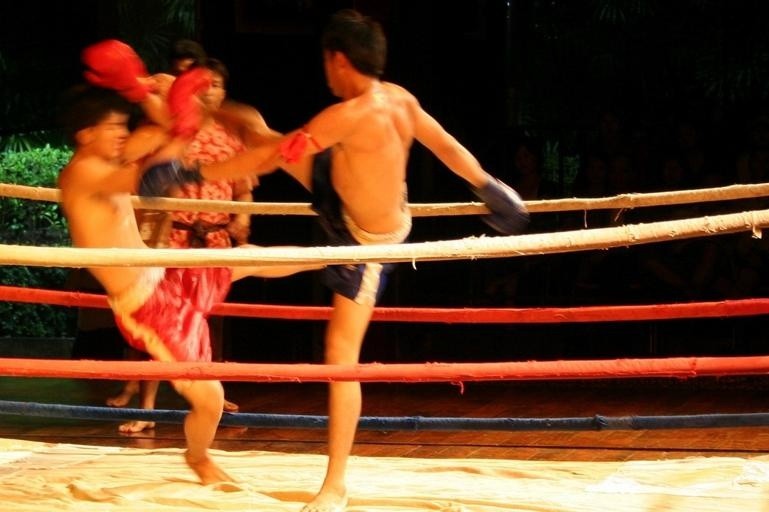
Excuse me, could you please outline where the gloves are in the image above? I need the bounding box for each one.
[79,37,160,104]
[471,178,532,235]
[139,158,204,197]
[168,67,215,138]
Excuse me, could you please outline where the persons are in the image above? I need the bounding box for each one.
[118,53,266,435]
[53,38,332,496]
[134,7,531,512]
[103,37,206,408]
